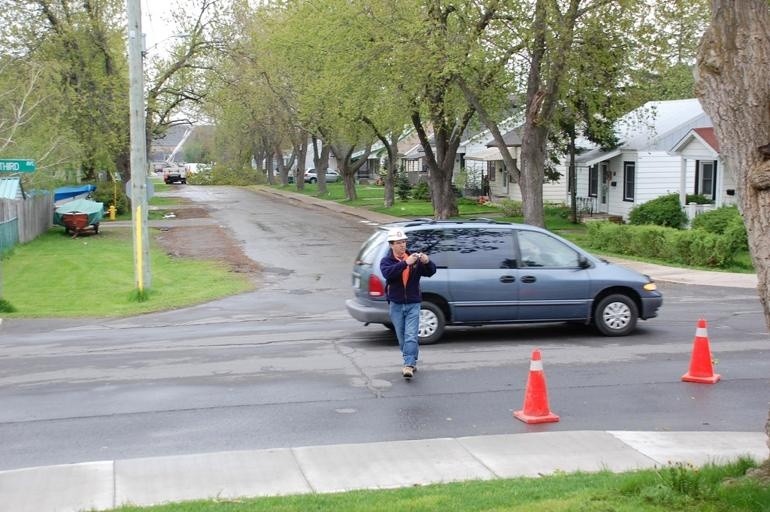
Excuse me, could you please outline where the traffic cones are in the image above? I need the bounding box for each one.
[682,316,723,388]
[512,348,561,425]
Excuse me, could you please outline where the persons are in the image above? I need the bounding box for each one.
[379,228,437,379]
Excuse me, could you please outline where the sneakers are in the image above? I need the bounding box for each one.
[401,365,417,378]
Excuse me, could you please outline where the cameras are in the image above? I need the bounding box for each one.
[415,254,422,260]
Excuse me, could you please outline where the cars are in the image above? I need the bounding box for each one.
[343,217,662,345]
[303,166,342,184]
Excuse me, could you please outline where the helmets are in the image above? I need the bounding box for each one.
[386,228,408,242]
